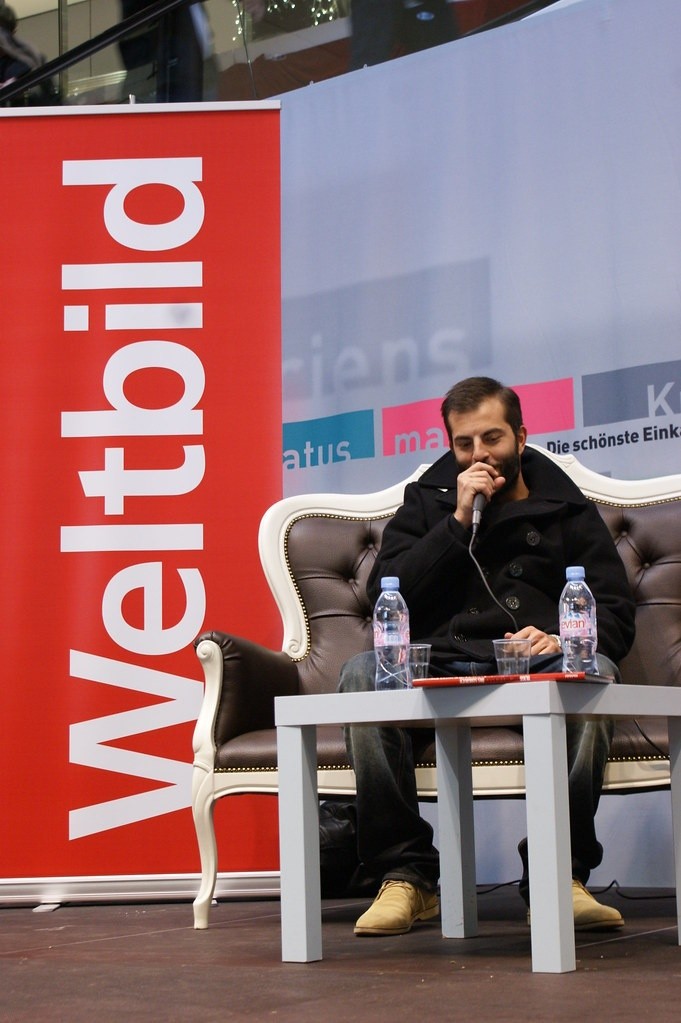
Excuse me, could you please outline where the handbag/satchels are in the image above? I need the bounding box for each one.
[315,797,380,898]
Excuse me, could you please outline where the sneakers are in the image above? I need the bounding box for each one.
[527,877,625,931]
[353,878,440,934]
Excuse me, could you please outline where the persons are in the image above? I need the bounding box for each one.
[119,0,217,104]
[350,0,459,71]
[337,376,636,936]
[0,0,63,107]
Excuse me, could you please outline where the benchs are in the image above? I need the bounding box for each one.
[191,442,681,934]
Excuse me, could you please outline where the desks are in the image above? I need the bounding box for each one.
[275,681,681,975]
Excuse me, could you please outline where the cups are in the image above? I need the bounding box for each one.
[409,644,433,679]
[492,639,531,675]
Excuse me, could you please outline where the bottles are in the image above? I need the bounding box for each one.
[375,576,411,692]
[559,566,600,676]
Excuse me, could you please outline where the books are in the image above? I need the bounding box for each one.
[412,672,586,687]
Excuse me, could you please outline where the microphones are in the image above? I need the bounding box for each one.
[472,493,486,531]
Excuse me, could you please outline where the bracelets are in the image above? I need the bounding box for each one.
[550,634,562,648]
